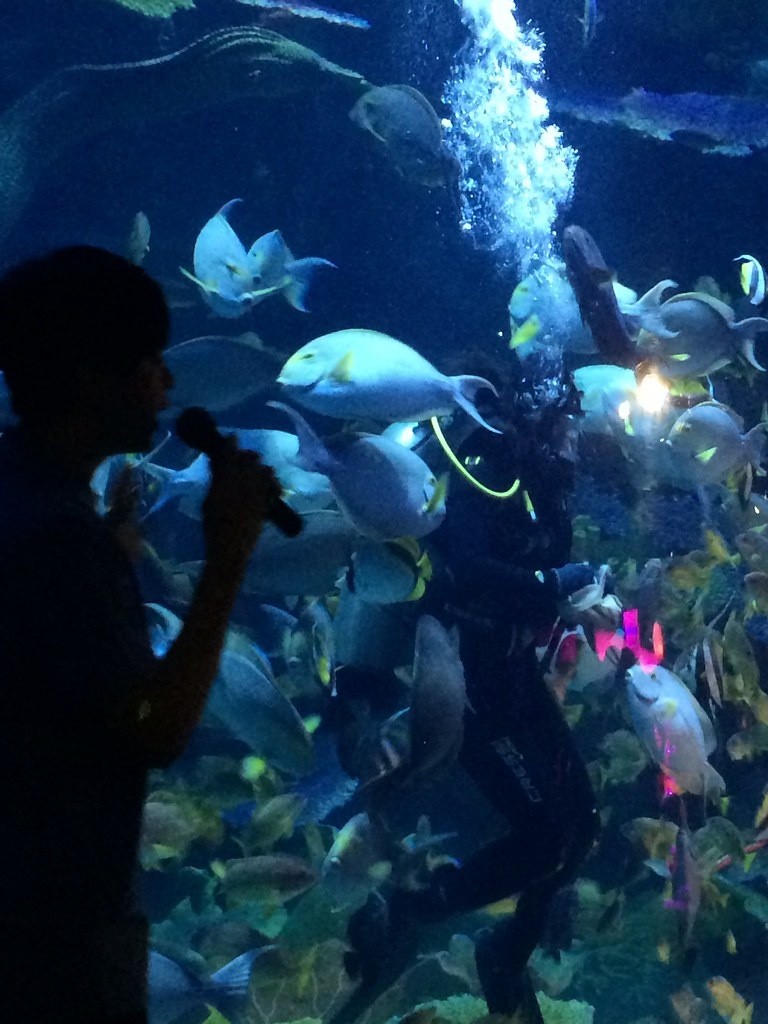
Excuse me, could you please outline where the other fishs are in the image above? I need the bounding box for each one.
[128,211,151,267]
[506,255,768,380]
[91,399,767,1024]
[347,84,463,187]
[177,198,339,321]
[274,328,505,436]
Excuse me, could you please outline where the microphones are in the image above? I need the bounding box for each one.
[176,406,305,539]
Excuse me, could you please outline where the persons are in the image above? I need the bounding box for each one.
[2,248,280,1024]
